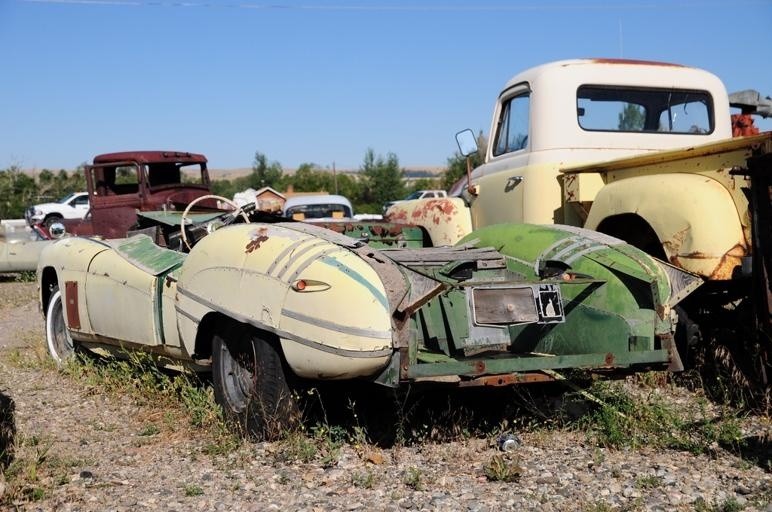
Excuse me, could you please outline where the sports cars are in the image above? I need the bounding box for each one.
[33,192,680,447]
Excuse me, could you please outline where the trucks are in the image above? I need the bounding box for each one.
[448,56,771,411]
